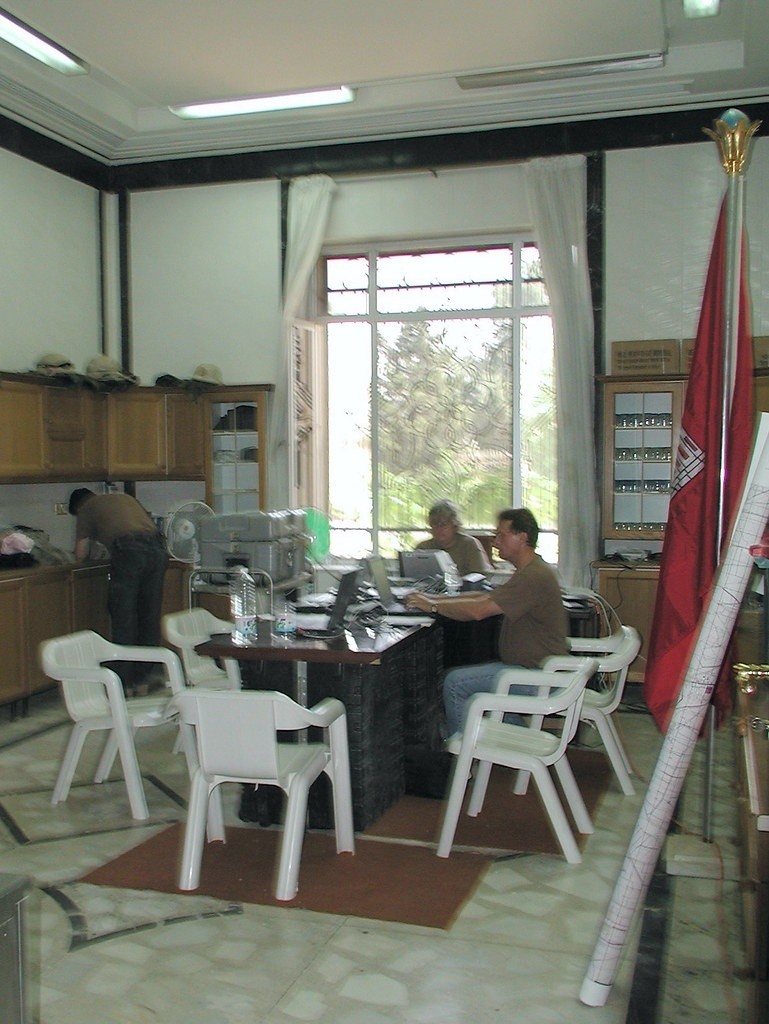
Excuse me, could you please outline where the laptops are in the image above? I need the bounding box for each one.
[402,551,443,580]
[294,568,364,634]
[364,553,436,618]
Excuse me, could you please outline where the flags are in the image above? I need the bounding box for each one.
[644,188,756,735]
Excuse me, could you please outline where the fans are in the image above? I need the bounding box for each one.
[165,501,220,587]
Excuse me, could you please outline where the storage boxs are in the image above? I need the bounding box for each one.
[610,338,697,375]
[752,335,769,369]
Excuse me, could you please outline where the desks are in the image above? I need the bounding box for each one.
[192,613,439,833]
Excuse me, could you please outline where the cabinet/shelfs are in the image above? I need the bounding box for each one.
[595,375,769,684]
[1,550,186,705]
[0,371,276,517]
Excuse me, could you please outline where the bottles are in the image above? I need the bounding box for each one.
[445,563,462,596]
[230,567,256,646]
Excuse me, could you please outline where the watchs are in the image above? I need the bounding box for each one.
[431,599,440,615]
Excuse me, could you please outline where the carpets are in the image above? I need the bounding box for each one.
[360,746,614,859]
[73,823,495,931]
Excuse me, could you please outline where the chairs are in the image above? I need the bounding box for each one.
[40,606,643,901]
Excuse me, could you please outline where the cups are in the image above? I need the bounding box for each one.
[615,413,670,491]
[275,601,296,633]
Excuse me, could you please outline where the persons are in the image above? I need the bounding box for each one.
[411,499,492,576]
[69,487,167,697]
[403,510,572,781]
